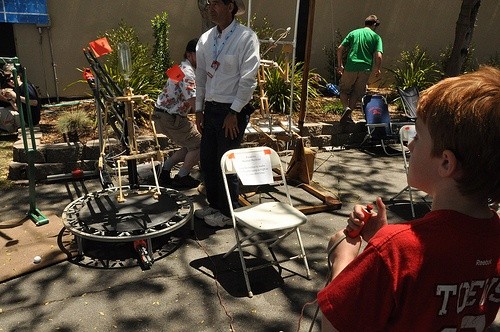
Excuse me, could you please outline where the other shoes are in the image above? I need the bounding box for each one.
[347,119,355,124]
[158,171,174,184]
[339,107,351,124]
[173,175,200,186]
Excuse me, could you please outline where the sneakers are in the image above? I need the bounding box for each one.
[205,212,235,227]
[195,207,218,219]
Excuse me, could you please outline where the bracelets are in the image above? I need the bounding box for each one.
[8,99,12,101]
[230,110,236,114]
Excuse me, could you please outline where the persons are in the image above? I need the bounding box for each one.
[152,38,202,190]
[194,0,260,226]
[0,64,41,132]
[336,14,383,124]
[317,66,500,332]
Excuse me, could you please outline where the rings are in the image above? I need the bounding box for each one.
[233,129,235,130]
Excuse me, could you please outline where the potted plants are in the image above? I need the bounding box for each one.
[56,108,93,142]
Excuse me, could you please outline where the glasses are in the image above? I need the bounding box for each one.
[6,77,14,83]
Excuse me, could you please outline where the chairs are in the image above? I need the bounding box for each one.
[362,86,433,220]
[220,147,311,298]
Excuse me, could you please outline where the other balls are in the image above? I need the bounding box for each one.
[34,256,41,263]
